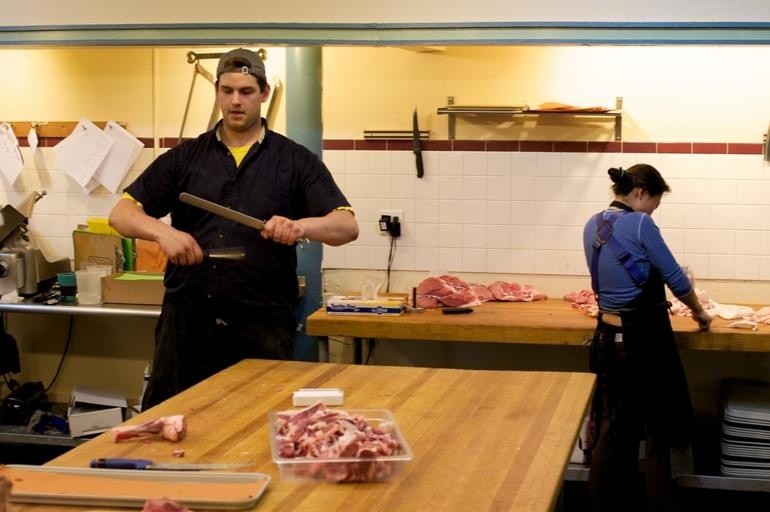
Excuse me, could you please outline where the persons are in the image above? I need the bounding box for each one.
[108,47,359,413]
[583,164,712,511]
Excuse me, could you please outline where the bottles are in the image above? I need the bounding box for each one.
[216,48,267,82]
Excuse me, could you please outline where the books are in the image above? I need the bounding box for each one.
[69,221,128,282]
[64,381,129,441]
[99,265,167,308]
[265,404,417,486]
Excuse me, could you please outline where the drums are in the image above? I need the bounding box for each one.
[411,104,426,180]
[88,455,260,472]
[178,192,309,250]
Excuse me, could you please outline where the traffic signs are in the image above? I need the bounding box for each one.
[377,209,405,237]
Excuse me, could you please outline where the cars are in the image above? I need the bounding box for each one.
[56,263,113,306]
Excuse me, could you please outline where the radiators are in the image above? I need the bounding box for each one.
[443,307,473,314]
